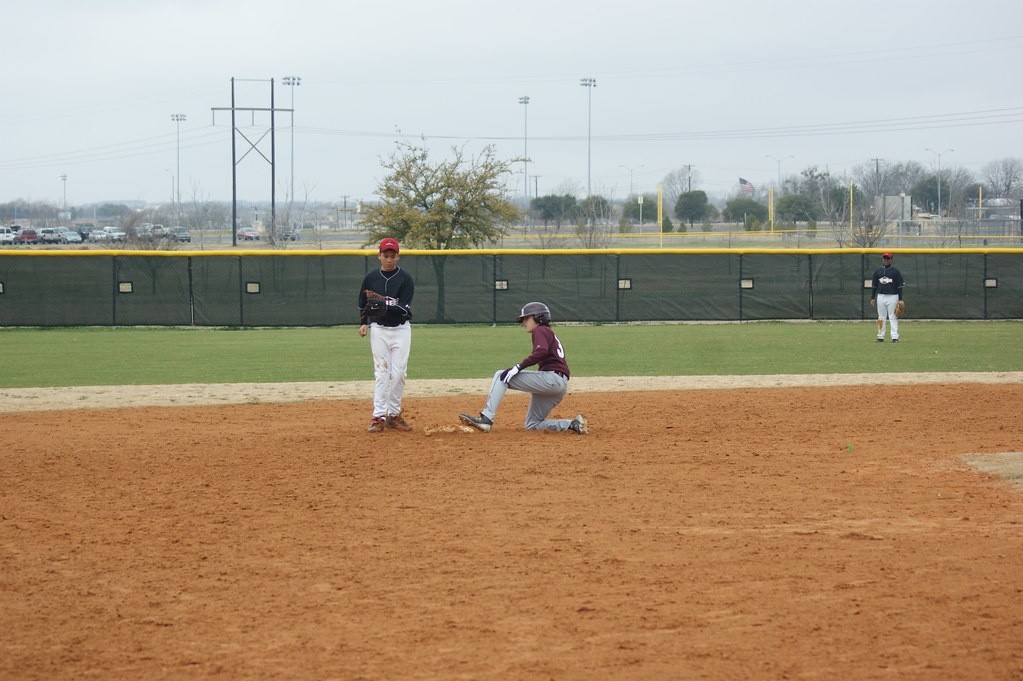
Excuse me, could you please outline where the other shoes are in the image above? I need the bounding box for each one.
[875,339,884,343]
[368,417,384,432]
[459,413,493,432]
[892,339,898,342]
[570,414,587,434]
[385,416,412,431]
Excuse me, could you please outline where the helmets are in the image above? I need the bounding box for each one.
[519,302,551,324]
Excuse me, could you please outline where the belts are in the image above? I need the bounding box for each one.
[555,371,569,380]
[377,321,404,326]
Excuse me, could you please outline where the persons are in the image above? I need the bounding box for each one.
[459,302,588,434]
[871,252,905,343]
[358,238,414,433]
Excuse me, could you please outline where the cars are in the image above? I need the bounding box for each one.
[276,226,296,241]
[169,227,191,242]
[0,224,168,245]
[236,228,260,240]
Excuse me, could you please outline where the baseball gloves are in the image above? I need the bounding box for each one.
[895,300,906,318]
[361,288,392,318]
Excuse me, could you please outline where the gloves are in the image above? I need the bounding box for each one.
[500,364,523,383]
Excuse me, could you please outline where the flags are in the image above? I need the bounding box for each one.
[739,178,755,193]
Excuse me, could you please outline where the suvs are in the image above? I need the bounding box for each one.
[12,227,38,245]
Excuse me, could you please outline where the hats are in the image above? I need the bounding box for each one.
[883,252,893,258]
[380,238,399,253]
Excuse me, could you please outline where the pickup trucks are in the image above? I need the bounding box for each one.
[37,228,59,244]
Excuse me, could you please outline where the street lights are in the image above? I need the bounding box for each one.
[282,75,301,202]
[165,169,174,209]
[618,164,644,225]
[580,77,597,198]
[518,97,530,197]
[925,148,955,221]
[172,114,186,203]
[765,155,794,187]
